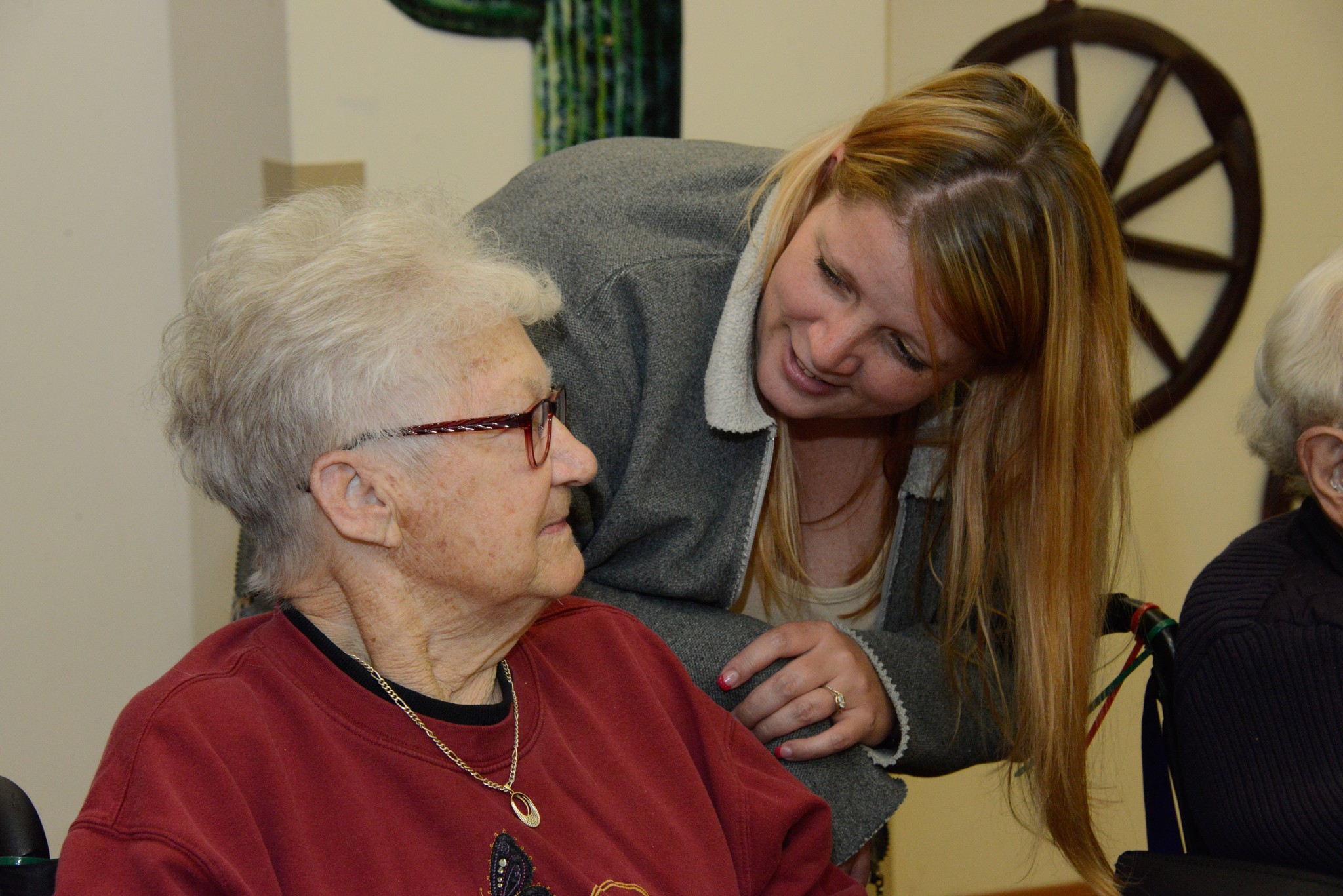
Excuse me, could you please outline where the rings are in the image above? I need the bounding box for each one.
[821,683,846,716]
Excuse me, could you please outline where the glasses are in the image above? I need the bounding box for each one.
[296,386,568,493]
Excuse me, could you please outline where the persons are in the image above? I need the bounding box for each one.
[1166,242,1343,896]
[387,58,1138,896]
[40,186,872,896]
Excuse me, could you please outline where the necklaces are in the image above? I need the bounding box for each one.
[337,642,546,830]
